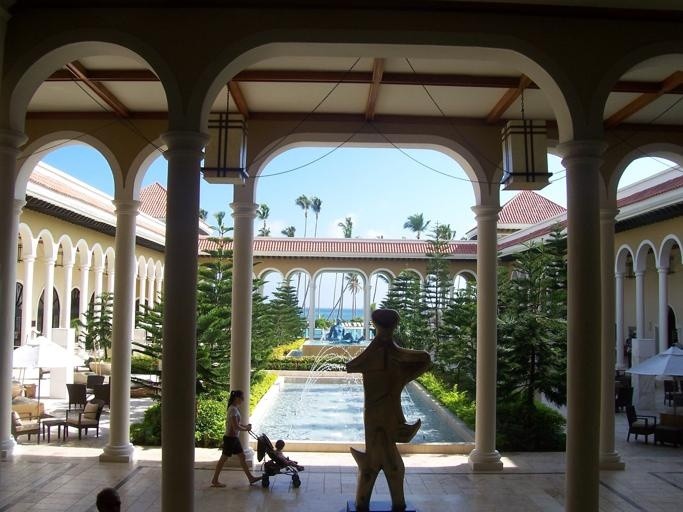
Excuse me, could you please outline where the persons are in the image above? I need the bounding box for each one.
[273,439,304,471]
[210,390,262,488]
[325,317,364,343]
[94,487,121,512]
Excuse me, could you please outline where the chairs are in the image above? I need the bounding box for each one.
[612,353,682,449]
[6,358,111,446]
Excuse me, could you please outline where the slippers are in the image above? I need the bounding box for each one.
[210,482,226,488]
[249,477,262,484]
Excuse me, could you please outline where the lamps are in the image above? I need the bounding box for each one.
[201,76,249,188]
[497,84,553,193]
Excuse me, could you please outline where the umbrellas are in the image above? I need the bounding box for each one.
[623,345,683,416]
[12,335,85,435]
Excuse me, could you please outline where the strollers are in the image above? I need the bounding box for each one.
[248,424,301,488]
